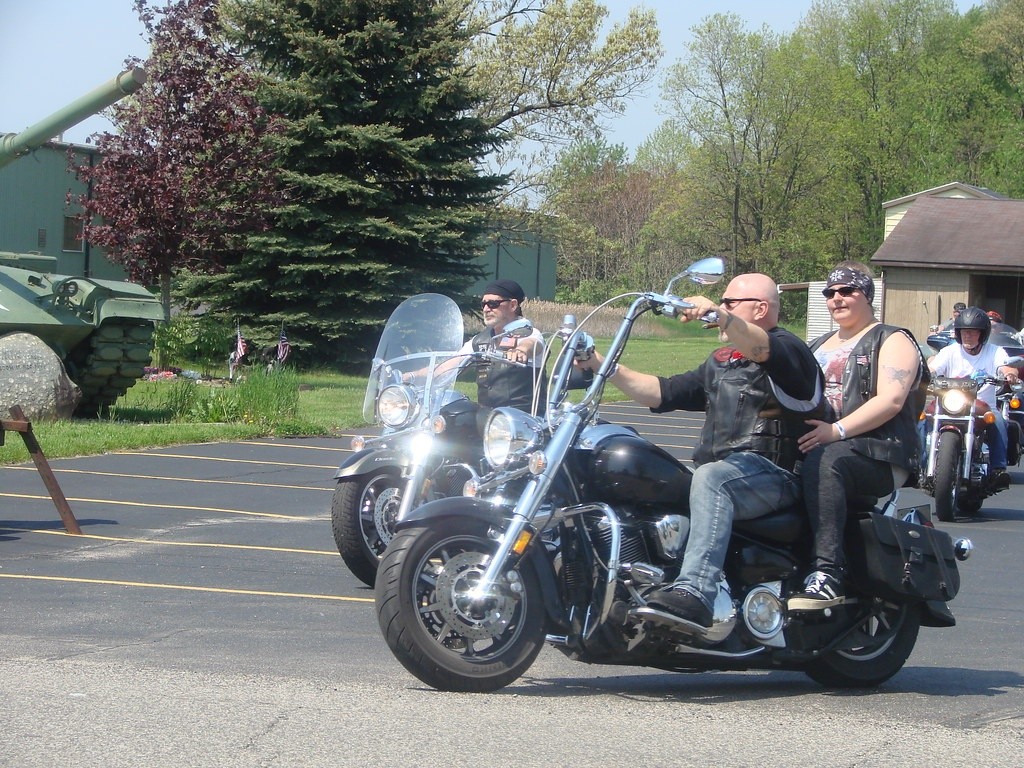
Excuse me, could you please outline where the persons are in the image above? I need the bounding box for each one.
[572,274,826,636]
[400,279,548,420]
[919,303,1024,489]
[787,262,930,611]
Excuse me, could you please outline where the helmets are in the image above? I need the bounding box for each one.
[986,311,1003,323]
[954,306,991,345]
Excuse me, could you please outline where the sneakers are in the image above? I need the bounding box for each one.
[787,571,845,611]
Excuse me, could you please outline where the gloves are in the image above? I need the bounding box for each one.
[507,347,528,364]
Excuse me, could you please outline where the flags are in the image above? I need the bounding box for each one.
[235,317,244,364]
[277,318,290,368]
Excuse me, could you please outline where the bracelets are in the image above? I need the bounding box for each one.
[510,348,525,354]
[406,371,415,384]
[833,421,846,441]
[605,362,619,382]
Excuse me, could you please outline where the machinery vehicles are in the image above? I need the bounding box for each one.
[0,67,165,418]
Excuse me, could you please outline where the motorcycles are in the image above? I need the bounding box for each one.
[918,319,1024,522]
[373,256,974,690]
[331,292,561,587]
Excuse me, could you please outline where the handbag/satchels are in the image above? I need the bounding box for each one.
[858,491,960,603]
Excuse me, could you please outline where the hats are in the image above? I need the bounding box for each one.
[482,279,525,304]
[954,303,966,311]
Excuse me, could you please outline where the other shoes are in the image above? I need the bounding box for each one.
[989,469,1011,489]
[647,588,713,628]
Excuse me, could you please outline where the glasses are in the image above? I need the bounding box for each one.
[822,287,859,299]
[720,298,761,307]
[480,299,511,309]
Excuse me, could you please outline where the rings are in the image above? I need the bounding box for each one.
[815,442,820,447]
